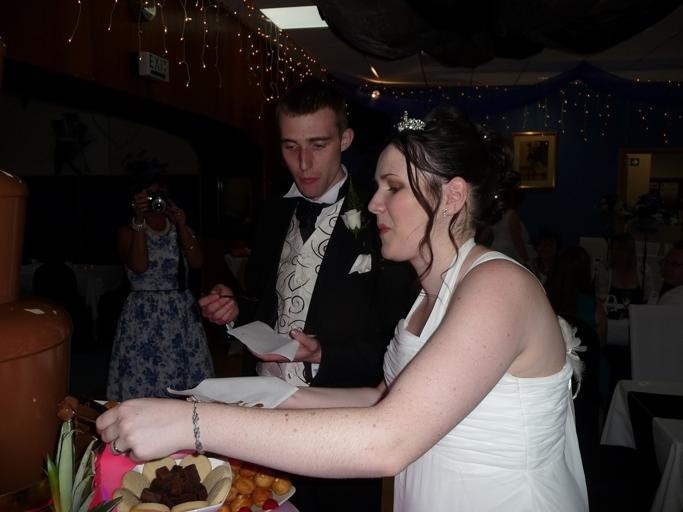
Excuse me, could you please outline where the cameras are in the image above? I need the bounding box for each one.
[146,192,168,214]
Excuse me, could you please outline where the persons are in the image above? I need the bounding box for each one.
[97,105,590,512]
[106,179,215,401]
[199,88,411,510]
[478,177,683,424]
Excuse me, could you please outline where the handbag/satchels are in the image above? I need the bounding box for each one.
[188,267,227,339]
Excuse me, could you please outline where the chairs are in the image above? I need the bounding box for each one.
[629,302,683,379]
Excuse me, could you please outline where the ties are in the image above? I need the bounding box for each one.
[294,180,346,244]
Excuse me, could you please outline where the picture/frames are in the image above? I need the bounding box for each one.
[511,130,557,189]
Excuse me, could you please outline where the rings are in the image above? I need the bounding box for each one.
[110,441,121,457]
[175,208,180,215]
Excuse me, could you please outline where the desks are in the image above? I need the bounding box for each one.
[600,381,683,512]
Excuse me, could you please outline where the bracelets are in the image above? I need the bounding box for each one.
[131,217,146,232]
[193,401,203,454]
[183,240,197,251]
